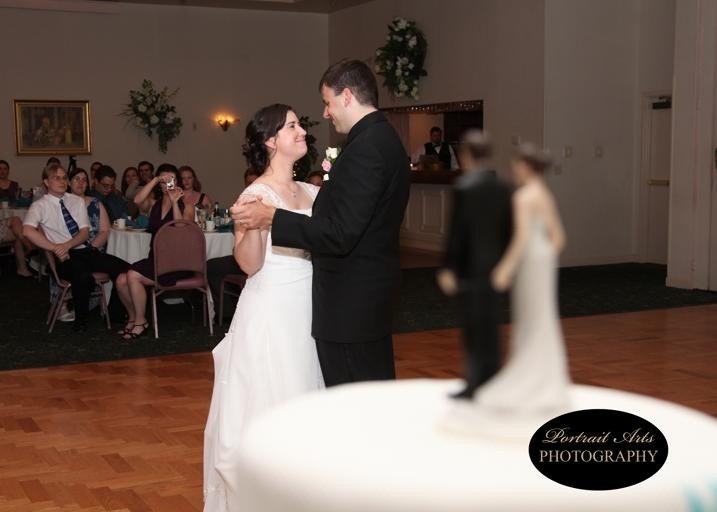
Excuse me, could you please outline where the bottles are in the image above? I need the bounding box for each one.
[193,199,231,231]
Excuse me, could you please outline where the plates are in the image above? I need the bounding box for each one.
[126,226,147,232]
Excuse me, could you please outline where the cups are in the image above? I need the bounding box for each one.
[114,219,126,228]
[1,201,8,208]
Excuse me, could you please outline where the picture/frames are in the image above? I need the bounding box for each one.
[12,98,93,155]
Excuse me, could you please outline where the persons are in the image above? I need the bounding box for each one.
[417,126,456,170]
[203,105,325,512]
[1,157,326,343]
[231,59,414,385]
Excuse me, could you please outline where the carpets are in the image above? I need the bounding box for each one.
[0,261,717,370]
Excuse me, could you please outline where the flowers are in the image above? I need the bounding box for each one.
[321,145,342,173]
[370,13,428,101]
[113,77,184,154]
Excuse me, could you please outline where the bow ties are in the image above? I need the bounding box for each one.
[433,144,442,147]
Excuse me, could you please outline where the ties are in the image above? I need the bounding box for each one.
[59,198,90,247]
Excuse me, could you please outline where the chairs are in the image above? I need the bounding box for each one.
[37,226,111,333]
[146,218,215,340]
[219,273,248,327]
[0,241,16,271]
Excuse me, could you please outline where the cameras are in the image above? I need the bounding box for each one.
[166,178,175,190]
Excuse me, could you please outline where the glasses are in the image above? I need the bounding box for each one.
[50,175,67,181]
[71,177,88,183]
[100,182,114,188]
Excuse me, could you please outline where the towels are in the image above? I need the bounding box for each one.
[121,212,134,226]
[0,196,9,202]
[216,220,234,232]
[134,215,150,228]
[14,200,32,206]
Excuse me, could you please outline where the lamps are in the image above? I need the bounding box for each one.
[218,120,230,131]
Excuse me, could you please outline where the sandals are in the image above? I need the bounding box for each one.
[56,302,75,322]
[117,318,149,340]
